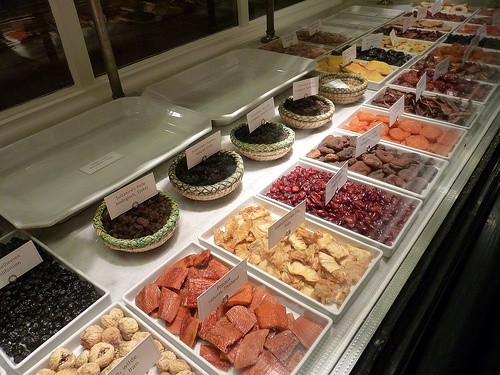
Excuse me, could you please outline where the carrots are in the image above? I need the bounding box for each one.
[342,111,461,157]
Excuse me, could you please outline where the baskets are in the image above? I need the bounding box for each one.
[92,182,179,254]
[230,120,295,162]
[316,72,368,104]
[168,147,244,202]
[278,93,335,129]
